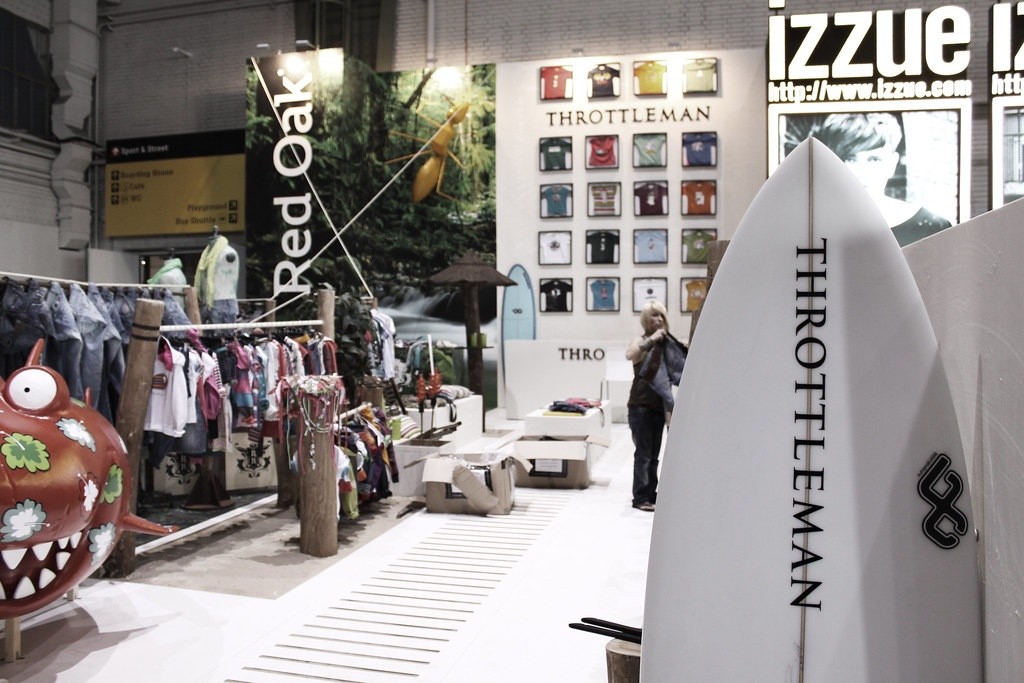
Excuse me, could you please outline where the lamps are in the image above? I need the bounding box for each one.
[296,39,316,52]
[257,43,275,57]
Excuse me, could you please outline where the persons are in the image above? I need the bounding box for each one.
[160,257,187,307]
[820,112,951,248]
[626,302,673,512]
[194,236,240,314]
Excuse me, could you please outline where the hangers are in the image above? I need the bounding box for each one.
[160,319,323,353]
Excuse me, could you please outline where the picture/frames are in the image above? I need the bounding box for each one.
[680,131,717,167]
[584,276,708,313]
[586,61,622,97]
[585,134,618,168]
[633,179,670,216]
[679,228,718,264]
[538,136,573,169]
[633,228,669,263]
[632,133,668,168]
[538,231,574,265]
[633,60,668,95]
[767,98,970,248]
[540,65,572,100]
[539,184,574,218]
[585,228,621,265]
[991,94,1024,210]
[681,58,719,95]
[588,181,622,217]
[681,178,718,215]
[539,278,575,313]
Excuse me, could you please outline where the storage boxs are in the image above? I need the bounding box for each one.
[402,453,533,515]
[497,431,612,490]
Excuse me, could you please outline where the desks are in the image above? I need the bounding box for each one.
[398,394,483,443]
[524,401,613,436]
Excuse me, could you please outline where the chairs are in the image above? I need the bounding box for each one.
[597,348,636,412]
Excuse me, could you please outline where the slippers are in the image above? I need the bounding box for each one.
[632,500,655,512]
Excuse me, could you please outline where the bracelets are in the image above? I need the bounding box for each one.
[639,339,654,350]
